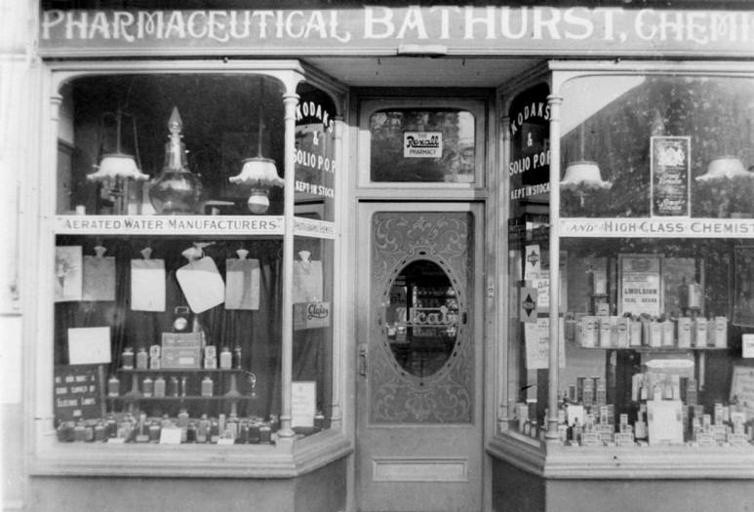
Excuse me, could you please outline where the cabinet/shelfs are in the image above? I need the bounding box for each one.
[486,61,754,459]
[30,55,350,472]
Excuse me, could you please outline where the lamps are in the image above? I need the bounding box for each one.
[228,107,286,214]
[694,82,748,183]
[86,108,148,181]
[558,78,614,208]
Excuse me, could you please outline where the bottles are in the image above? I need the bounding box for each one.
[57,342,279,444]
[523,312,754,446]
